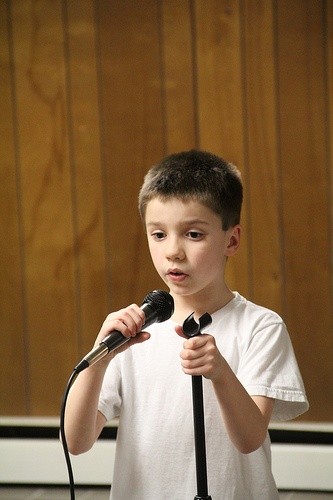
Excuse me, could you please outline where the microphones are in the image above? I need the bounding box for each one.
[73,289,176,375]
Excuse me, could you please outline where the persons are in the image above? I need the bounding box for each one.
[59,147,310,500]
